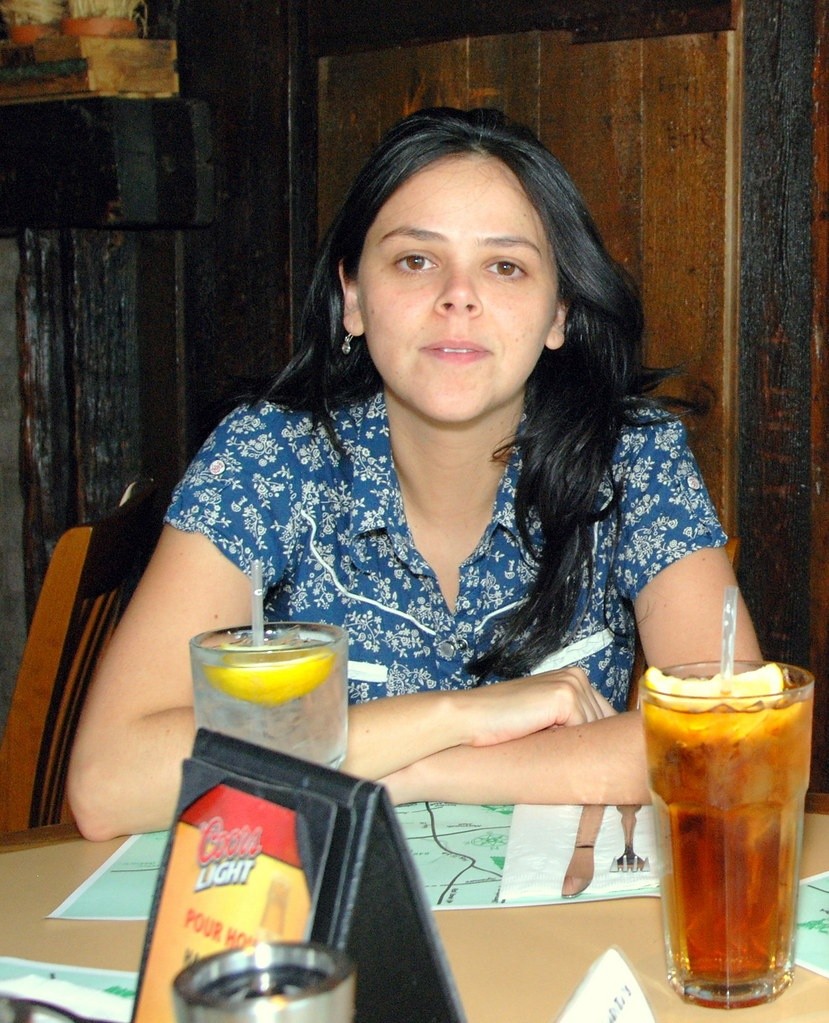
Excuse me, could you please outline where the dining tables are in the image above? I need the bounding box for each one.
[0,786,829,1023]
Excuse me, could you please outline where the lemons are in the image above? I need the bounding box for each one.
[202,643,336,704]
[642,663,784,743]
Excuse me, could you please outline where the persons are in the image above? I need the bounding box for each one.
[66,106,764,841]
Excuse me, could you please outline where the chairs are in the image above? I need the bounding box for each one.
[0,475,179,834]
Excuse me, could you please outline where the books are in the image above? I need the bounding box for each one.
[0,36,180,106]
